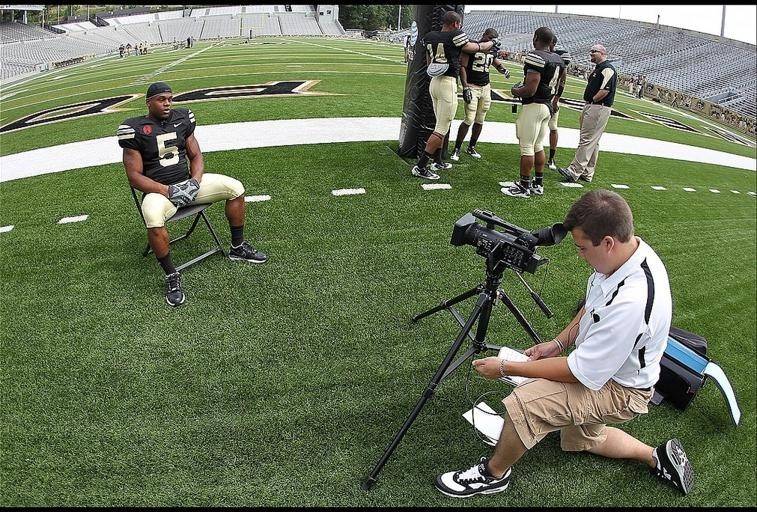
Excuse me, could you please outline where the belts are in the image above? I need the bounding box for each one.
[586,101,611,107]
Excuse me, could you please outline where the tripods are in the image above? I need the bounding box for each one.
[358,287,542,490]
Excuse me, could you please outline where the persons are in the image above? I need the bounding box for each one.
[450,28,510,162]
[435,189,695,497]
[501,27,565,197]
[548,35,565,171]
[116,82,268,307]
[570,67,756,139]
[559,44,617,183]
[411,12,500,180]
[112,37,196,59]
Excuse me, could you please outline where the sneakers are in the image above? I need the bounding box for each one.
[430,161,453,171]
[450,147,460,161]
[529,176,543,195]
[558,167,575,182]
[433,457,512,499]
[580,174,590,182]
[165,271,185,307]
[229,241,266,264]
[652,438,695,495]
[467,145,481,159]
[411,166,440,180]
[547,158,557,171]
[500,180,531,199]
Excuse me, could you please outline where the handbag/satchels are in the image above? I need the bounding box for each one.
[577,297,741,428]
[426,63,449,78]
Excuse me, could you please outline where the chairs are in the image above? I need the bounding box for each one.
[123,159,227,274]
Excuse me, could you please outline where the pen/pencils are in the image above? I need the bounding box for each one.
[503,376,511,380]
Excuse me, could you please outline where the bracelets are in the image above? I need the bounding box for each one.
[554,337,565,352]
[500,359,504,380]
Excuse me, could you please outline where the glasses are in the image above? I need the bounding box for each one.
[589,50,600,53]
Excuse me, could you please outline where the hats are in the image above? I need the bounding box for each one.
[146,82,172,98]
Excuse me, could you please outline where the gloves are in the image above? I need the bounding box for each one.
[496,64,511,79]
[168,184,192,207]
[180,178,200,201]
[492,37,502,46]
[462,86,472,104]
